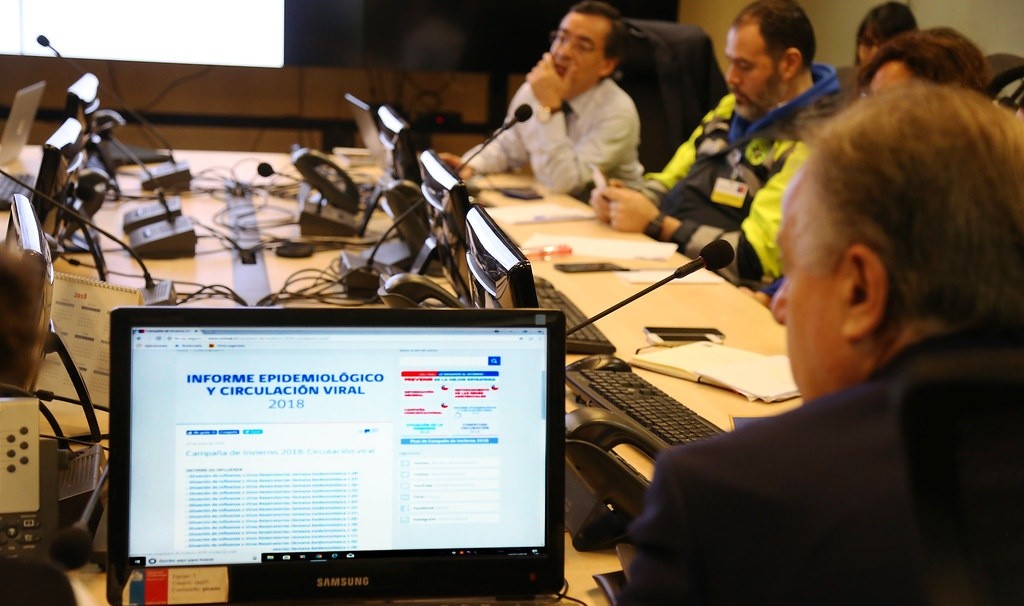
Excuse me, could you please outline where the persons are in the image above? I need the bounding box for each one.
[855,2,917,67]
[437,1,644,205]
[626,83,1024,606]
[739,27,989,325]
[588,0,842,286]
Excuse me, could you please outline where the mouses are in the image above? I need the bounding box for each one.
[533,277,554,289]
[566,355,633,372]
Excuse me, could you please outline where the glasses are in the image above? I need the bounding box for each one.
[550,32,608,59]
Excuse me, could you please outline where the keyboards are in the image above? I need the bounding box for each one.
[535,286,616,356]
[567,366,726,453]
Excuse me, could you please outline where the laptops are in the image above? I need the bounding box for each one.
[0,79,47,168]
[347,95,387,170]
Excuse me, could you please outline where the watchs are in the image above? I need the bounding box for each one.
[536,107,563,123]
[645,213,664,239]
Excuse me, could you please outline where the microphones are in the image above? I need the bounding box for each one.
[98,107,197,260]
[336,104,533,297]
[256,162,306,187]
[566,240,736,336]
[36,34,190,190]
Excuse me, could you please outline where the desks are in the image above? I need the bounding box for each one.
[0,145,804,606]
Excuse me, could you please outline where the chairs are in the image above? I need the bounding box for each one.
[613,20,728,176]
[981,53,1024,101]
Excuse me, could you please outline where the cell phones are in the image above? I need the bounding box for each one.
[644,327,725,347]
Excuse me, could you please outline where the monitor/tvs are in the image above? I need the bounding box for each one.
[111,105,566,606]
[0,73,100,400]
